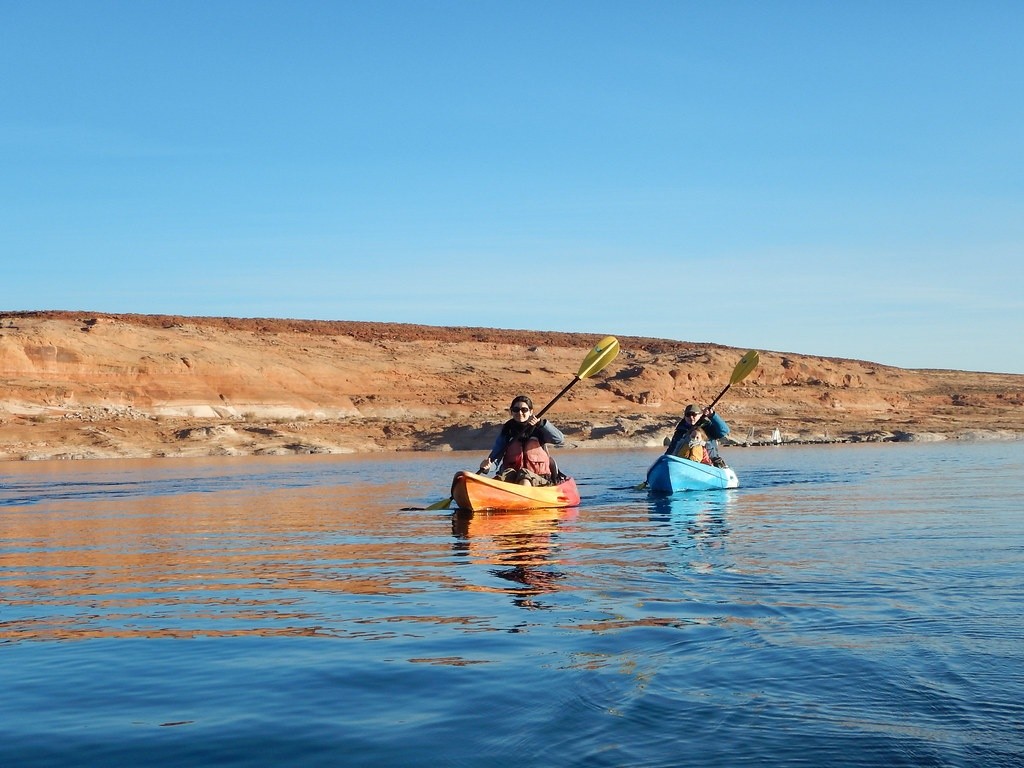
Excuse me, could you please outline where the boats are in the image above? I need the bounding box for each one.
[644,453,741,492]
[449,465,582,512]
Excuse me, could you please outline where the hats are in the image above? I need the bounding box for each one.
[684,405,700,414]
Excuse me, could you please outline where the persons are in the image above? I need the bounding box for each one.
[664,404,730,466]
[678,427,713,465]
[480,396,564,486]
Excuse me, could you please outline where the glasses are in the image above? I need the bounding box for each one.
[512,407,531,413]
[685,413,701,418]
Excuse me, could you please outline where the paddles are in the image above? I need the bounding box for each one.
[426,335,619,512]
[635,350,759,491]
